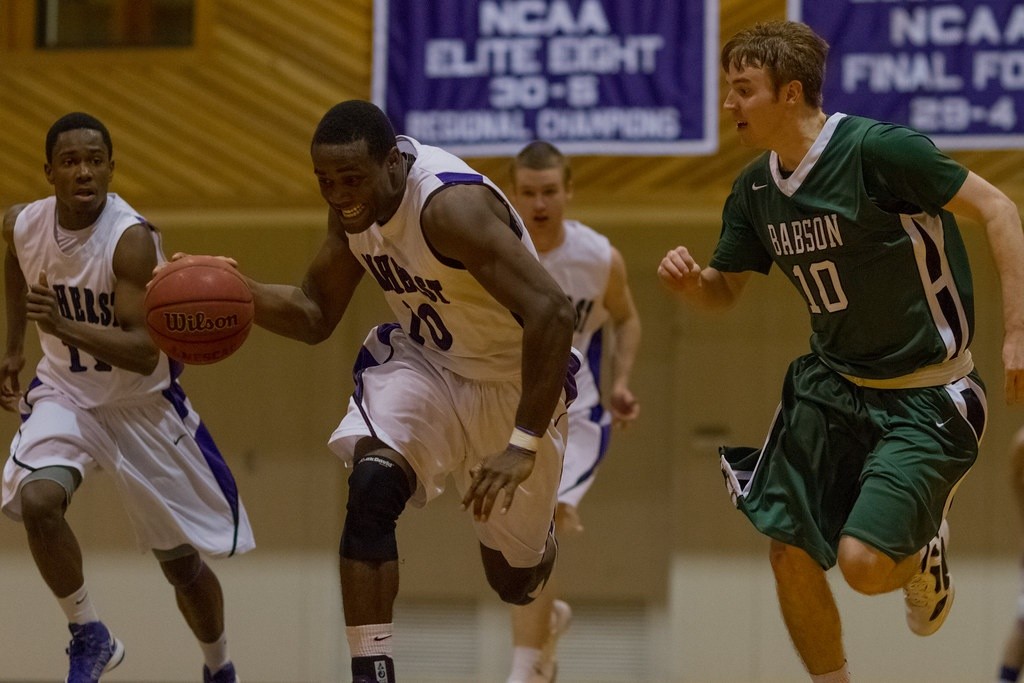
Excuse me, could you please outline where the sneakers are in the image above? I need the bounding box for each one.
[64,621,125,683]
[905,519,955,637]
[204,661,239,683]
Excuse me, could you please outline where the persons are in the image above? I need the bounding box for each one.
[0,113,258,683]
[142,98,579,681]
[506,140,643,683]
[653,19,1024,682]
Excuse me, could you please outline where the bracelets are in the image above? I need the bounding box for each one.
[508,425,543,455]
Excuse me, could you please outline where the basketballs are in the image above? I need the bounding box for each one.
[141,252,256,369]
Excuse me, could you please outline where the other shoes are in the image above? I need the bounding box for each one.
[531,597,573,682]
